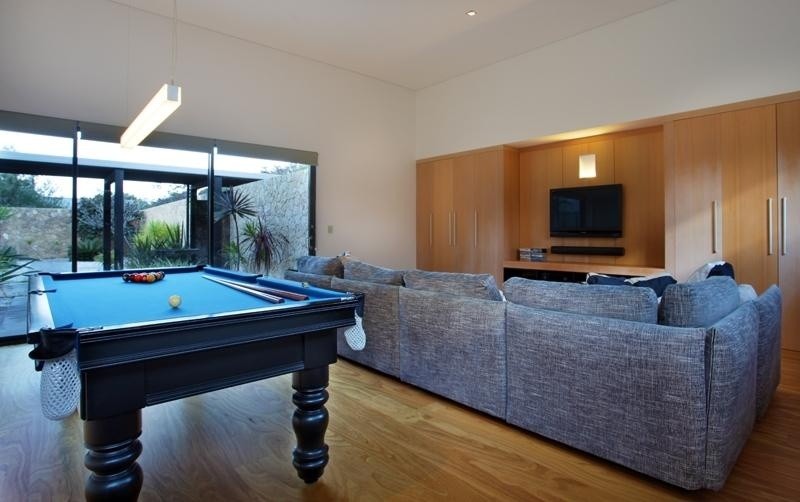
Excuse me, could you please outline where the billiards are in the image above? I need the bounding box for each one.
[122,271,165,283]
[168,295,182,307]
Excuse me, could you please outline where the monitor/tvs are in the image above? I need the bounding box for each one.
[549,184,624,238]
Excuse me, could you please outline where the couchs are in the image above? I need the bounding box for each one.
[284,251,782,492]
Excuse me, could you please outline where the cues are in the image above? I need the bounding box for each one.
[211,277,309,301]
[201,275,285,303]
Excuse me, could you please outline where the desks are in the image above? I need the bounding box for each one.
[24,264,365,502]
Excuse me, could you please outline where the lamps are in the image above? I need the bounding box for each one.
[120,0,182,151]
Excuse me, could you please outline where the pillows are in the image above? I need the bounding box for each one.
[581,270,679,297]
[338,255,403,286]
[659,275,741,328]
[294,251,347,278]
[403,268,503,301]
[738,283,758,302]
[685,260,735,282]
[501,275,660,324]
